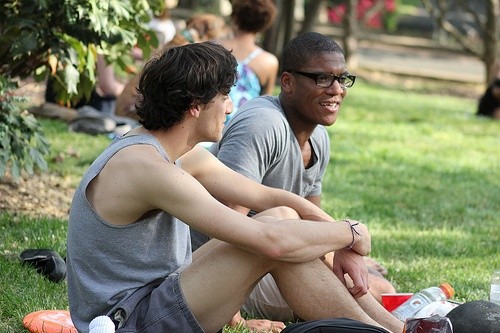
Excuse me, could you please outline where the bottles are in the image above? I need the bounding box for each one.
[490,272,500,306]
[391,282,453,320]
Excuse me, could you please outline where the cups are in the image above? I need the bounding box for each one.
[381,294,412,312]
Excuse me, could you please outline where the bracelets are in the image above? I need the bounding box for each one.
[342,220,361,250]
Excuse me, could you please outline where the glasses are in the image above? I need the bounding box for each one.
[293,71,356,88]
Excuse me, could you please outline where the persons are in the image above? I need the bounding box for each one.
[66,41,404,333]
[45,0,279,151]
[475,78,500,122]
[189,31,397,322]
